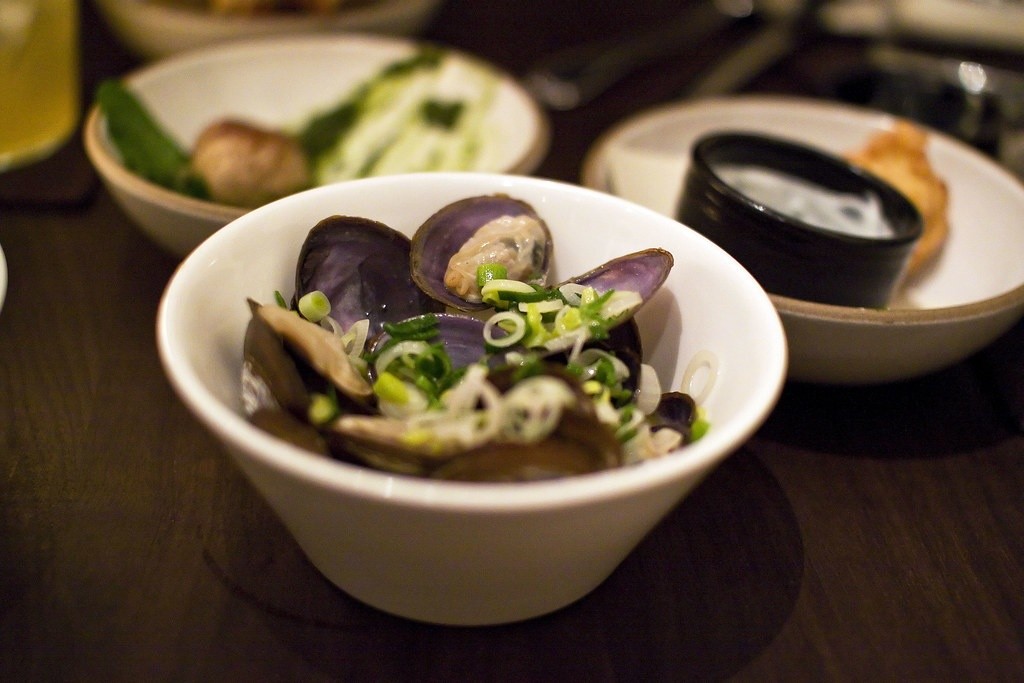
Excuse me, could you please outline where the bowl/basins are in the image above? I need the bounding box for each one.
[97,0,439,60]
[83,30,549,256]
[678,132,924,309]
[581,95,1022,384]
[154,172,789,627]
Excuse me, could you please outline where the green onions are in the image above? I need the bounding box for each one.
[281,262,709,464]
